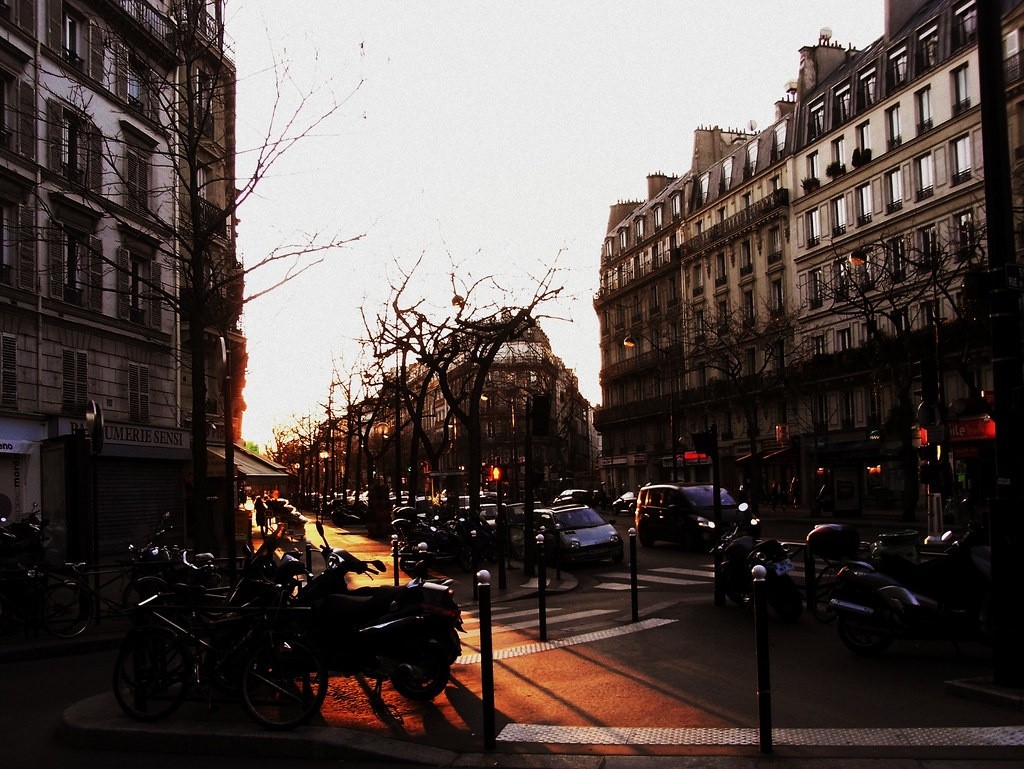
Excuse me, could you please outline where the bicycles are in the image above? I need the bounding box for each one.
[810,541,878,625]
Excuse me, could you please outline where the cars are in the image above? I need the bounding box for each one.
[535,503,625,571]
[611,490,639,516]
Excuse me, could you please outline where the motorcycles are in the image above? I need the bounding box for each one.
[1,472,611,731]
[701,501,804,627]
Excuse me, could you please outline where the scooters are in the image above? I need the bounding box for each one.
[803,512,992,658]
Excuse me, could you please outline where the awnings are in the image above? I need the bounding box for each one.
[207,442,288,476]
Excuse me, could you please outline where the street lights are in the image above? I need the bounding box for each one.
[623,333,678,483]
[850,244,951,497]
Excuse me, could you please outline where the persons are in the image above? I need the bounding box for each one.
[254,496,267,531]
[598,485,603,500]
[790,476,800,509]
[587,487,593,504]
[769,480,786,513]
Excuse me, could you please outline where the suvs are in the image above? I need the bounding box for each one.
[637,482,761,556]
